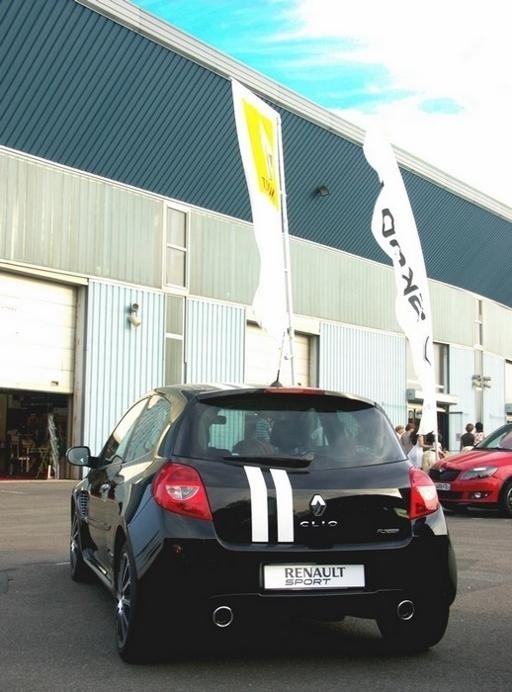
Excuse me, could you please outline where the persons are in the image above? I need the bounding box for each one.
[395,423,448,475]
[460,423,476,454]
[255,414,271,443]
[474,421,487,449]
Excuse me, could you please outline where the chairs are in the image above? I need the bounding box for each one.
[270,421,310,455]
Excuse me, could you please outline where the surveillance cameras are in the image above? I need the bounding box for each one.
[128,316,140,327]
[131,303,139,311]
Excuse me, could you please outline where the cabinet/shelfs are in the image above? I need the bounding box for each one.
[8,442,20,475]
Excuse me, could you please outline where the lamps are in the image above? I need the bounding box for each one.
[128,304,142,326]
[315,185,328,195]
[471,375,491,388]
